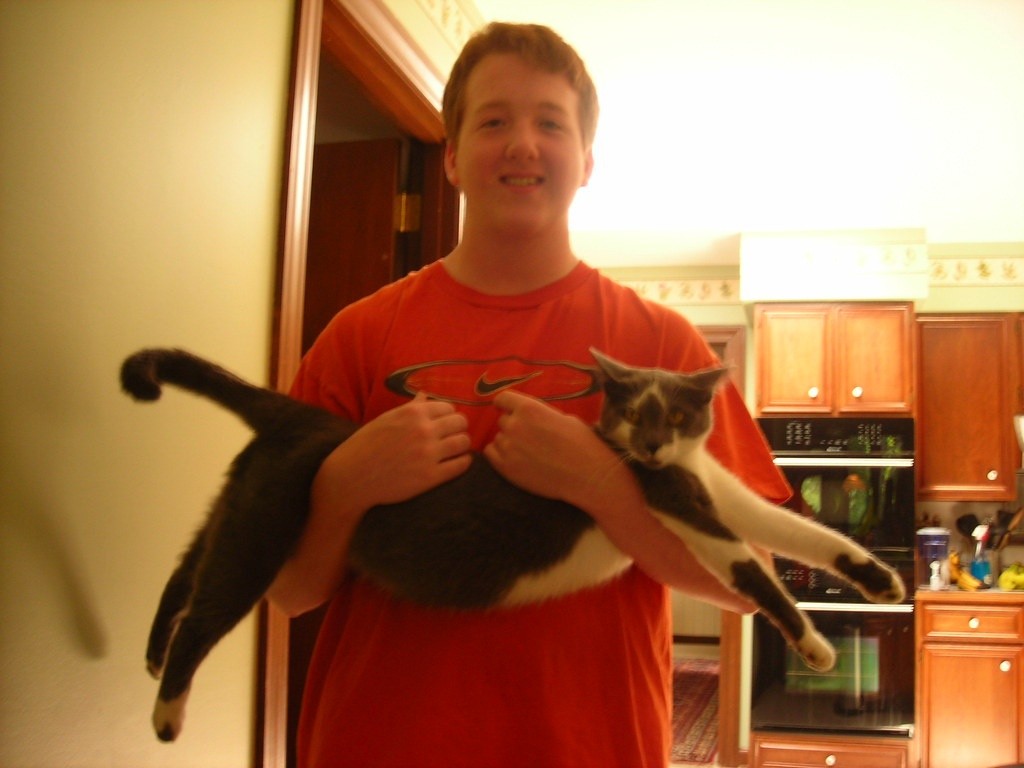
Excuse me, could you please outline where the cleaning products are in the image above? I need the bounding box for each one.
[970,525,992,589]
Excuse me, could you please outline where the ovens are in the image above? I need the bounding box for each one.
[751,417,917,735]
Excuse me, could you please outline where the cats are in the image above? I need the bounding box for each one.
[117,344,907,744]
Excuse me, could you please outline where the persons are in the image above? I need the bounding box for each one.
[264,21,793,768]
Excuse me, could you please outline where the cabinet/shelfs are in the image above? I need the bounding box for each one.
[915,588,1024,768]
[750,300,914,419]
[718,598,921,768]
[912,311,1024,502]
